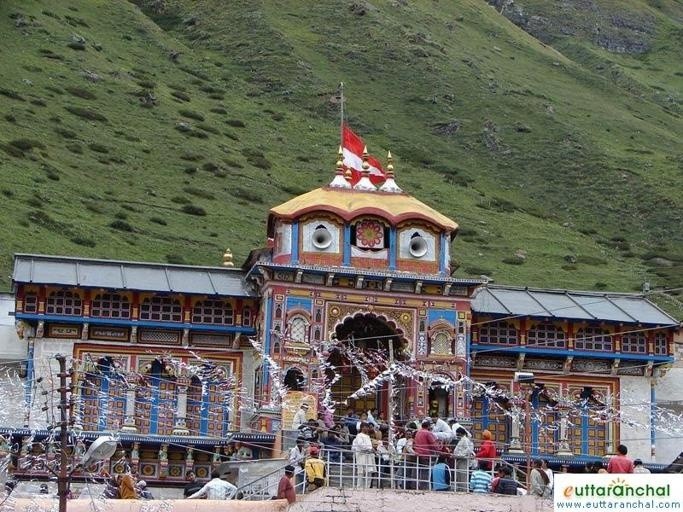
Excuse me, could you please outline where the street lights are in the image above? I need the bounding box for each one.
[54,352,119,512]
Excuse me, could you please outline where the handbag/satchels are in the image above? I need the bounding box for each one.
[313,478,325,488]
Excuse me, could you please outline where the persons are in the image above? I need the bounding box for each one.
[530,459,549,498]
[585,462,593,473]
[99,464,154,500]
[183,470,207,499]
[188,472,238,500]
[278,465,296,503]
[541,459,553,493]
[593,462,608,473]
[289,403,517,494]
[633,458,651,474]
[607,445,633,473]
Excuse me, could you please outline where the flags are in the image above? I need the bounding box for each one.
[343,128,386,183]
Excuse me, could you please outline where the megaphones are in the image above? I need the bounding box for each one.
[312,229,332,248]
[409,236,428,257]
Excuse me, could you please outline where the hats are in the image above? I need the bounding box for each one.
[309,447,320,455]
[300,403,310,411]
[482,430,491,440]
